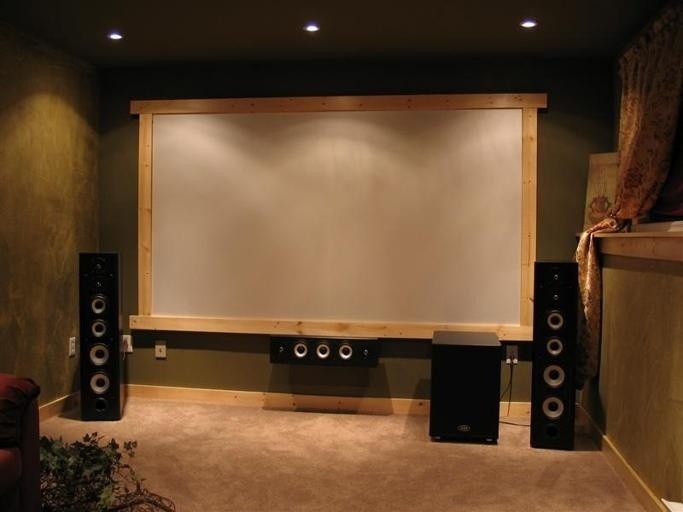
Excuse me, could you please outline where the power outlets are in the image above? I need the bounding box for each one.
[123,335,133,353]
[69,337,75,357]
[506,345,519,365]
[155,340,166,358]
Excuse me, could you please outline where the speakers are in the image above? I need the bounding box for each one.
[270,335,379,367]
[530,262,578,450]
[79,252,125,421]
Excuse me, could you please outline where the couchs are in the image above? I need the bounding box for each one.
[0,373,41,511]
